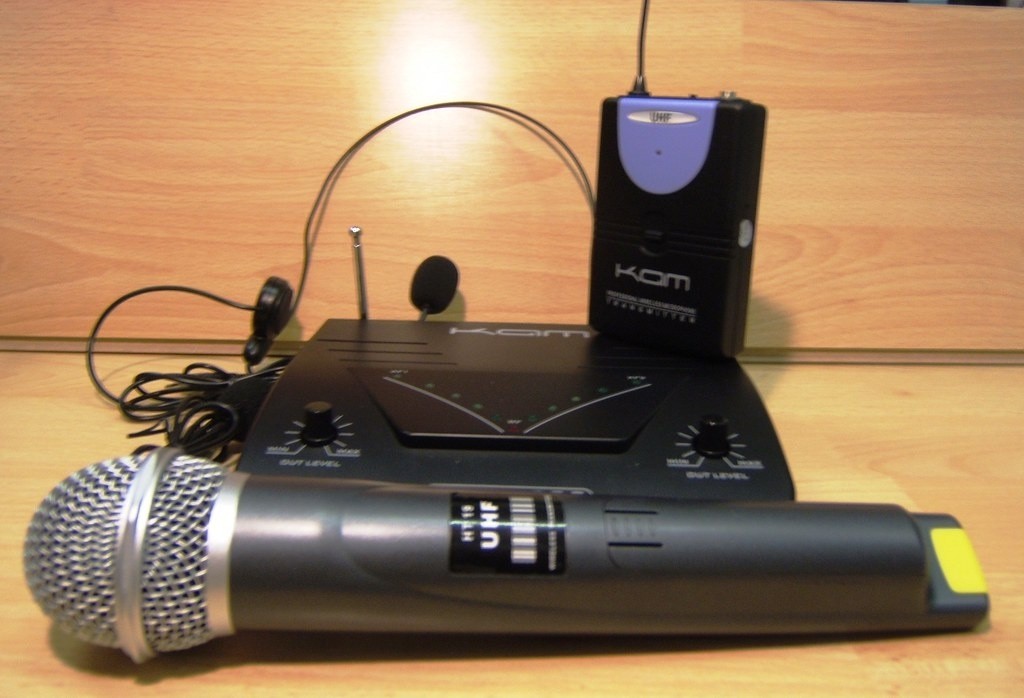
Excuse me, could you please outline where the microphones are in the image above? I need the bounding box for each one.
[409,254,457,321]
[22,442,985,665]
